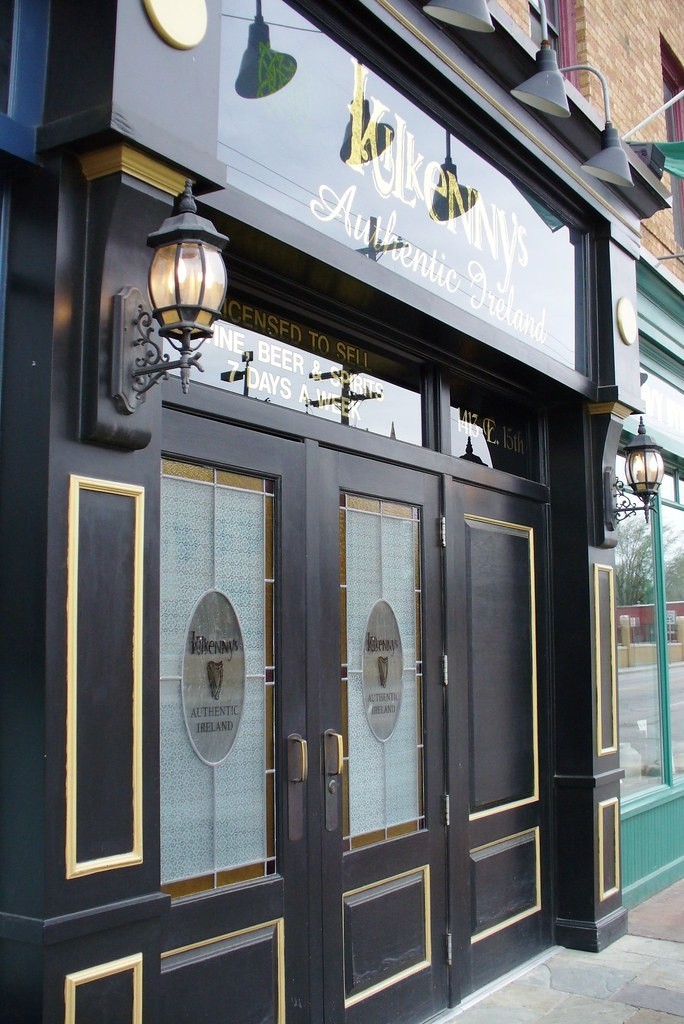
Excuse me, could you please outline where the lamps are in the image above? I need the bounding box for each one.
[604,415,664,532]
[339,60,394,165]
[422,0,497,31]
[509,0,570,118]
[429,129,479,222]
[559,64,635,188]
[106,173,229,416]
[235,0,298,98]
